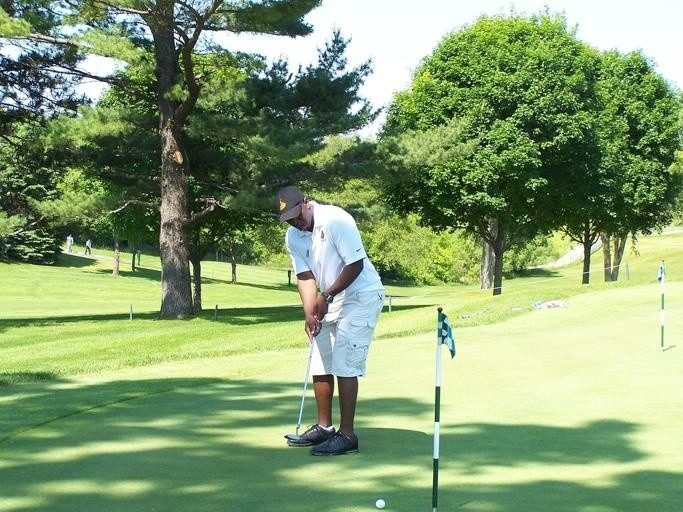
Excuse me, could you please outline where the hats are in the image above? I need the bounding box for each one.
[275,186,305,226]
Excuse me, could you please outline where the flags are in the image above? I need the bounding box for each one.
[657,262,666,281]
[437,313,456,359]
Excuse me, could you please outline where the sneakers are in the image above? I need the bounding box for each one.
[311,429,360,456]
[287,424,335,447]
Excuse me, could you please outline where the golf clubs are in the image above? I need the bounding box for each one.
[284,314,318,440]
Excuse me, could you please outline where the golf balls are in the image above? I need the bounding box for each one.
[376,499,385,509]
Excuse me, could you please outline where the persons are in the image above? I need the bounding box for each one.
[66,234,74,252]
[274,186,386,454]
[85,238,92,256]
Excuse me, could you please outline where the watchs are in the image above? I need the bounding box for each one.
[321,291,334,303]
